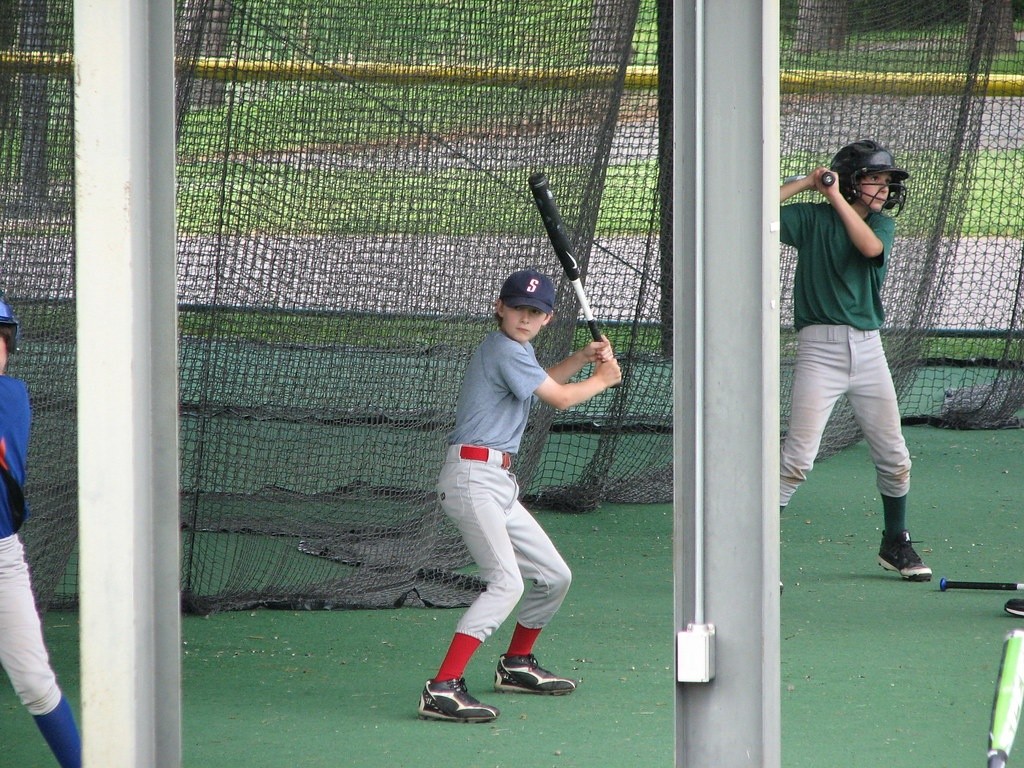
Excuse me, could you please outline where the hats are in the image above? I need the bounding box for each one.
[500,268,556,318]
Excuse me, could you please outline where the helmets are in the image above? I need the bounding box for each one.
[831,139,909,203]
[0,297,19,340]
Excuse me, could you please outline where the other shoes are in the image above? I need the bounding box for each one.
[1005,598,1024,618]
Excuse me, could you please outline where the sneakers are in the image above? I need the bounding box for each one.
[418,676,501,723]
[494,653,576,697]
[877,531,932,583]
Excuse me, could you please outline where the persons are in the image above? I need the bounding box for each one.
[1004,599,1024,616]
[0,301,81,768]
[780,140,933,588]
[418,269,621,723]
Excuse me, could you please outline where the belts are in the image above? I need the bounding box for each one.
[460,445,510,469]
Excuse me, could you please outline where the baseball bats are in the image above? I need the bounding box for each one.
[784,171,836,191]
[987,629,1024,768]
[527,172,622,388]
[940,577,1024,592]
